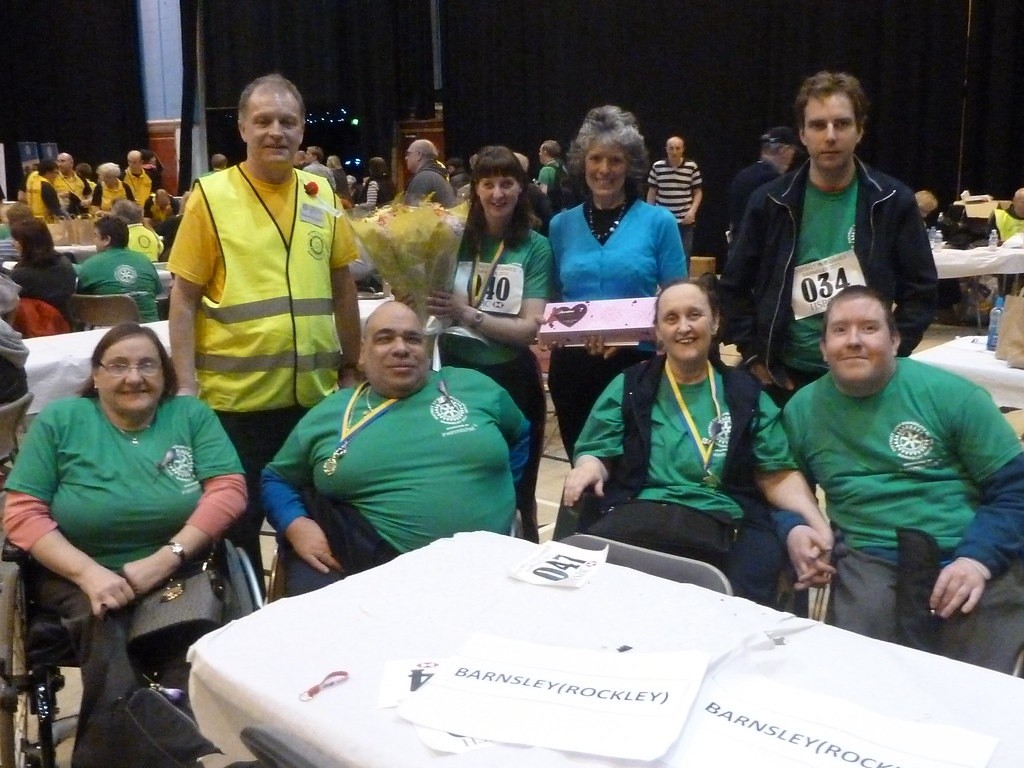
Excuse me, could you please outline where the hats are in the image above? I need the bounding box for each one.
[760,126,807,153]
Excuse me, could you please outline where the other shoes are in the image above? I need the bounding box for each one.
[935,310,961,325]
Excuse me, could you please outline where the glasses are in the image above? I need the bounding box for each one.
[405,151,419,156]
[97,360,163,377]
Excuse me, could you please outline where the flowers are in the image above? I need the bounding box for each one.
[350,190,474,370]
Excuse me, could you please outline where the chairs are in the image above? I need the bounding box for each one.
[0,292,1024,768]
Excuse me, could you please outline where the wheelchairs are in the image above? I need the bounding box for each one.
[0,539,264,767]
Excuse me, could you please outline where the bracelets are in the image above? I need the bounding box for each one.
[343,361,358,368]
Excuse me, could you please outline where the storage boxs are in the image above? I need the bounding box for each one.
[689,256,717,280]
[954,201,1013,225]
[540,297,657,345]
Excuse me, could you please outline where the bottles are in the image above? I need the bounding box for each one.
[986,298,1005,350]
[988,229,999,251]
[924,226,943,253]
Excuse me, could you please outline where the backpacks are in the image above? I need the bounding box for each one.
[545,163,582,213]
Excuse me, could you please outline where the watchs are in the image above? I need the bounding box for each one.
[470,309,484,328]
[164,541,186,566]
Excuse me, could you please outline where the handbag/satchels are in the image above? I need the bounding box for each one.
[121,688,223,768]
[895,525,944,652]
[586,502,732,561]
[996,288,1024,368]
[127,544,224,662]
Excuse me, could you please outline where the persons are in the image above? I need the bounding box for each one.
[164,73,363,608]
[112,199,164,262]
[91,149,164,219]
[293,138,471,209]
[259,299,531,573]
[0,203,76,339]
[719,70,939,506]
[143,189,191,262]
[914,187,1024,327]
[77,215,164,331]
[0,322,248,768]
[754,284,1024,676]
[563,281,835,610]
[199,154,227,178]
[0,152,97,223]
[646,126,805,272]
[446,139,569,237]
[397,146,553,544]
[535,106,690,469]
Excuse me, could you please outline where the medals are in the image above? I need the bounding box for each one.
[324,459,337,476]
[699,476,717,488]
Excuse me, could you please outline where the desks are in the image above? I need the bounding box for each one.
[907,336,1024,415]
[150,260,173,302]
[931,246,1024,334]
[23,319,171,421]
[54,245,98,262]
[187,528,1023,768]
